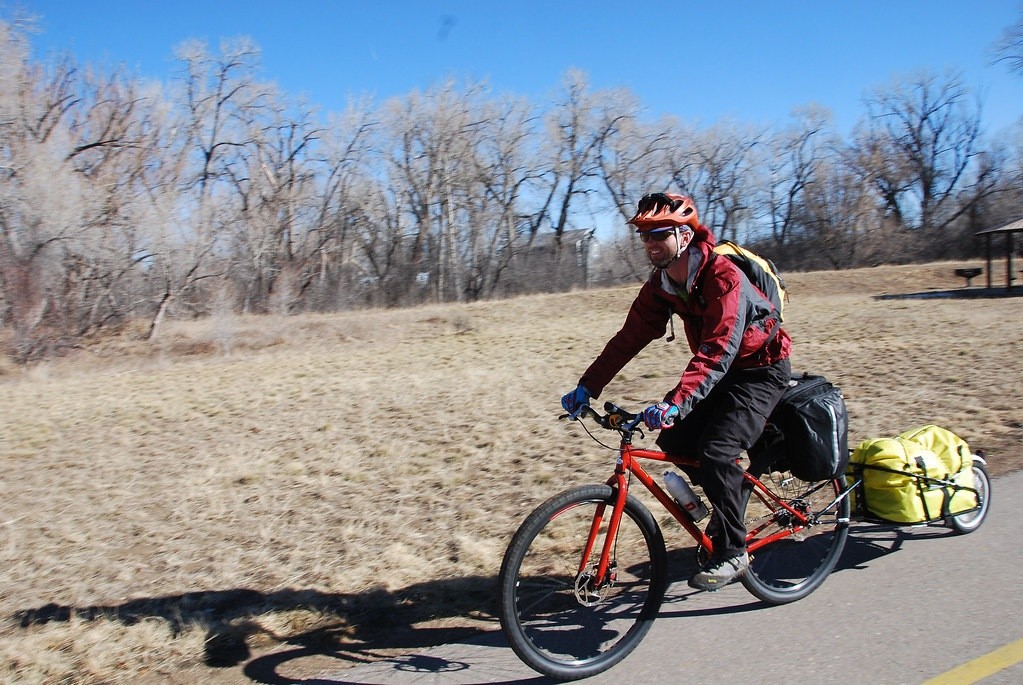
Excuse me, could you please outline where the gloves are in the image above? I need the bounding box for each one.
[644,401,680,431]
[561,385,592,418]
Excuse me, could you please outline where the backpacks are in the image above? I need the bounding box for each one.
[693,239,788,361]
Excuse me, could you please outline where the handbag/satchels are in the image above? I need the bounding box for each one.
[765,371,849,481]
[845,424,976,522]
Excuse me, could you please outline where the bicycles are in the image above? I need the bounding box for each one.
[498,401,862,680]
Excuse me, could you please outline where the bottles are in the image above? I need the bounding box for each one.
[664,471,706,520]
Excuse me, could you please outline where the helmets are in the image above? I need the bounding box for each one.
[625,193,699,231]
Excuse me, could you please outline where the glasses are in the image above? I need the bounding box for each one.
[640,230,676,242]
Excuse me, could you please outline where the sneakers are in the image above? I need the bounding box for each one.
[705,510,721,536]
[688,551,749,591]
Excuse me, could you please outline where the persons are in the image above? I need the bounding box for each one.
[561,193,792,590]
